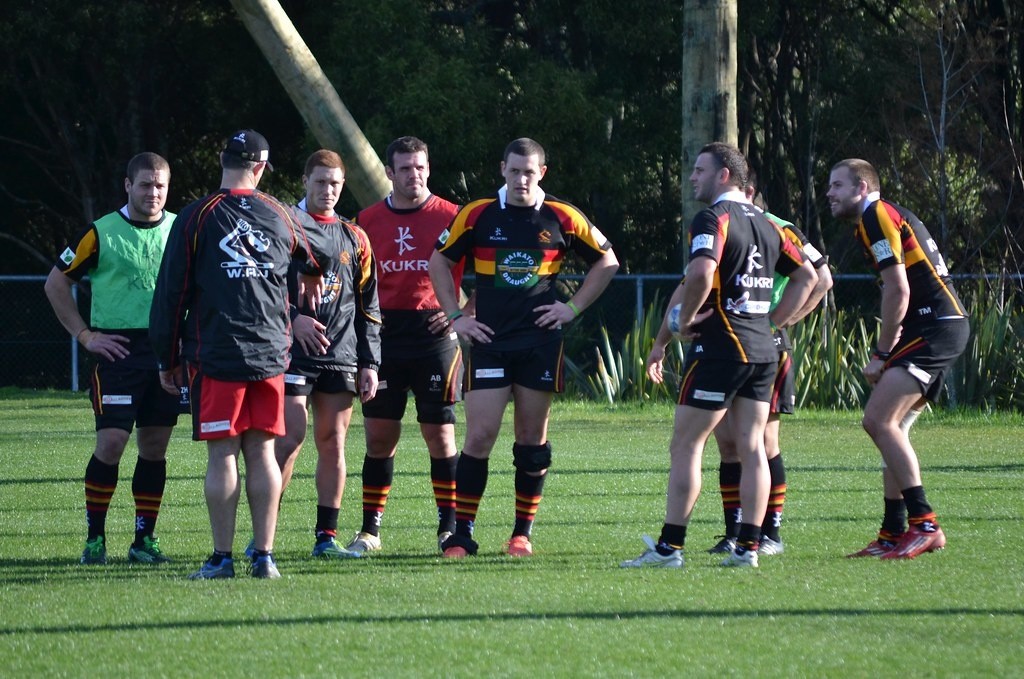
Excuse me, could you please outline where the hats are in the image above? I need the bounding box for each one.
[222,130,274,172]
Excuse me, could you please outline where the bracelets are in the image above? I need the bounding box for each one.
[770,319,777,335]
[567,301,579,316]
[76,328,89,339]
[447,310,463,324]
[872,348,889,361]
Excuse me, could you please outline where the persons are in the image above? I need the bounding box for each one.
[244,150,382,560]
[426,138,619,557]
[44,151,182,564]
[148,129,338,579]
[346,136,475,549]
[619,141,834,564]
[826,158,970,558]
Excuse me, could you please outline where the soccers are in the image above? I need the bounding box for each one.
[667,303,693,343]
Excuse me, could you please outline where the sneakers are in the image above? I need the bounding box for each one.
[81,536,107,566]
[345,532,382,552]
[311,537,361,559]
[719,549,759,568]
[128,536,172,565]
[704,535,737,554]
[756,535,784,556]
[879,524,946,560]
[188,556,235,580]
[501,535,534,557]
[437,532,453,555]
[619,535,684,568]
[845,529,906,559]
[245,555,282,580]
[443,546,467,558]
